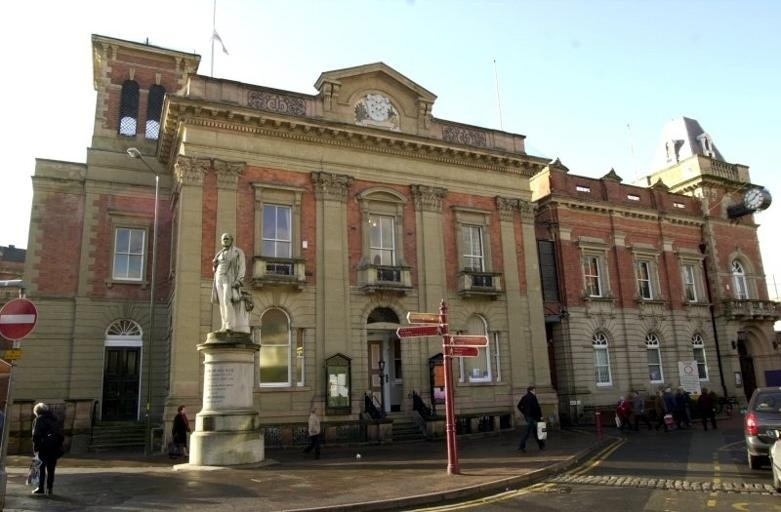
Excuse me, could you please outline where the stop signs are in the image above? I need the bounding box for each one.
[1,298,40,341]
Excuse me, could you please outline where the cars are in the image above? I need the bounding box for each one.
[768,436,781,489]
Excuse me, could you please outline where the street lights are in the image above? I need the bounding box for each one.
[378,358,388,416]
[125,145,160,459]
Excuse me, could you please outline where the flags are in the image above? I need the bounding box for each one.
[212,29,229,55]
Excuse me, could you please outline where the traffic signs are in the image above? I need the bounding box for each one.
[448,346,482,358]
[406,311,442,325]
[395,327,443,339]
[448,335,491,347]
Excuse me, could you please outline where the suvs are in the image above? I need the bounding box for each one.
[739,384,781,470]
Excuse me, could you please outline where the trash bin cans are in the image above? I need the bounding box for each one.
[151,428,162,452]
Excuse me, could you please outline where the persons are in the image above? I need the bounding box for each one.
[304,407,322,461]
[516,384,546,453]
[28,401,63,497]
[172,403,193,459]
[208,231,249,333]
[612,386,718,435]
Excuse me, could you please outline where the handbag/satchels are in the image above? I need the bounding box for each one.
[537,421,548,440]
[663,414,673,425]
[615,413,621,428]
